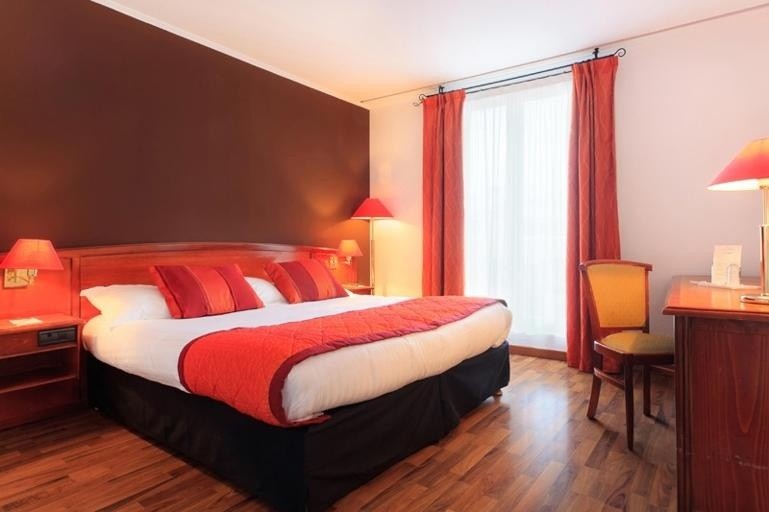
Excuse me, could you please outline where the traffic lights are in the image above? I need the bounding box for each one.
[80,258,350,330]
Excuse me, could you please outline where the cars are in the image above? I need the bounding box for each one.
[726,264,740,286]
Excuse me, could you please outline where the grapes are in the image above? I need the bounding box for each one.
[0,314,83,430]
[341,283,374,295]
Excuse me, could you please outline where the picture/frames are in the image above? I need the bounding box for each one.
[662,275,769,512]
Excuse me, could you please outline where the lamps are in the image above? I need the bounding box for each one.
[579,258,674,451]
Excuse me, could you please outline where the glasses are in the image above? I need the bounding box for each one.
[707,136,769,305]
[330,240,363,270]
[0,239,65,288]
[349,198,394,294]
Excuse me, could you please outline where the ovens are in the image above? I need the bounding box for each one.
[75,242,512,512]
